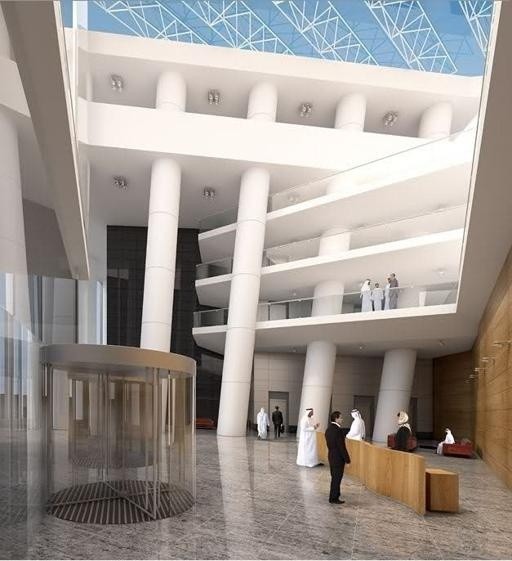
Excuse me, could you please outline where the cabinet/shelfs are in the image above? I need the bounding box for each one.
[424,468,459,514]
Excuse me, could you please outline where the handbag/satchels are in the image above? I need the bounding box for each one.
[281,425,284,433]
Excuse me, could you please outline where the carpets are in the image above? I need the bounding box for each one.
[44,480,194,525]
[70,449,156,469]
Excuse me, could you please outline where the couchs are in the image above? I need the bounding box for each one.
[188,417,217,430]
[442,439,474,459]
[387,434,417,452]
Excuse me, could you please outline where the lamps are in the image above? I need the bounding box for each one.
[201,188,216,202]
[382,113,397,129]
[298,103,311,119]
[108,74,123,93]
[207,88,220,106]
[112,177,126,194]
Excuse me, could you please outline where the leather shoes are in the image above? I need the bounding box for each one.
[329,498,344,505]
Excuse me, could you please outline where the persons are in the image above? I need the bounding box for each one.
[435,428,456,456]
[383,278,390,310]
[295,408,320,468]
[388,272,399,309]
[371,282,384,311]
[271,406,283,440]
[391,411,412,453]
[324,410,351,505]
[257,408,270,441]
[359,279,373,313]
[345,408,366,441]
